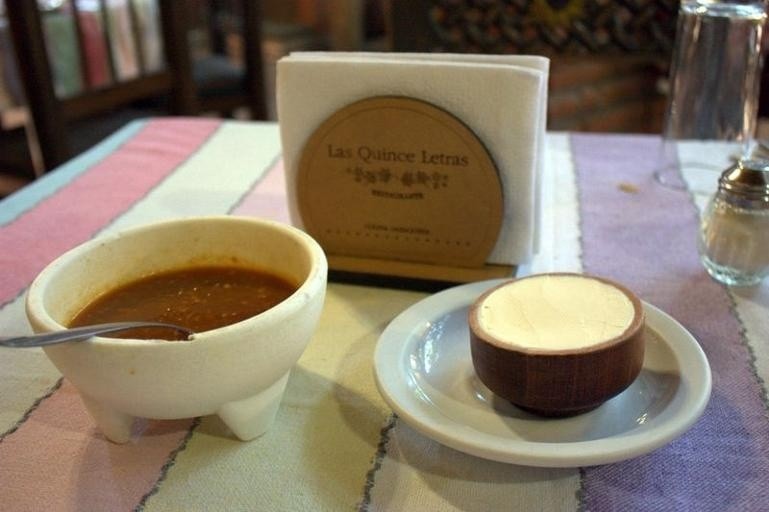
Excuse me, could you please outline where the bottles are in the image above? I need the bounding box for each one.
[701,160,769,285]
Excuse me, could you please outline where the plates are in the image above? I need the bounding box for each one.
[372,278,713,466]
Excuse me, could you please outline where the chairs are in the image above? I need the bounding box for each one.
[181,0,269,122]
[0,0,187,196]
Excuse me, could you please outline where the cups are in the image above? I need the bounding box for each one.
[655,0,768,192]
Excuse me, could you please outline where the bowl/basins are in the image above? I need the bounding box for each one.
[25,214,329,444]
[470,273,644,419]
